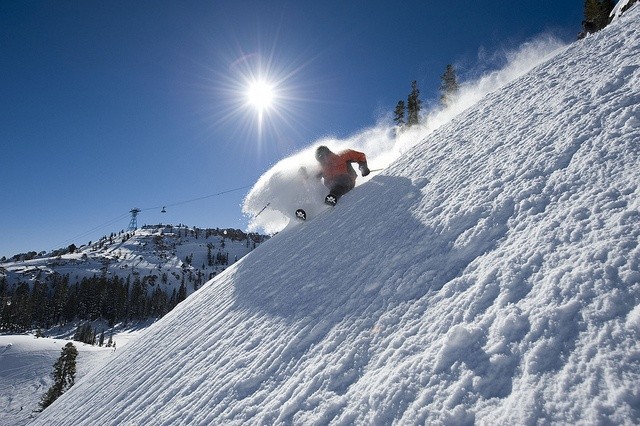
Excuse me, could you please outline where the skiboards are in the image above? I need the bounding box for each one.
[295,194,337,221]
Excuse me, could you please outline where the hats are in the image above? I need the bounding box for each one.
[315,146,330,160]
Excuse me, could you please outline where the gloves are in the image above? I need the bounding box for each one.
[359,161,370,176]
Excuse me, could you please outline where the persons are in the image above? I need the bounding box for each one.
[312,146,370,207]
